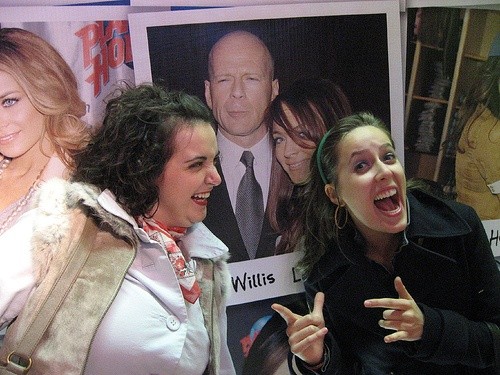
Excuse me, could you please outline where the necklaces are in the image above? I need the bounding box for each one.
[0,156,46,235]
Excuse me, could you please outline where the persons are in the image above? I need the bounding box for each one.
[195,29,352,263]
[0,58,499,375]
[0,28,99,335]
[241,298,314,375]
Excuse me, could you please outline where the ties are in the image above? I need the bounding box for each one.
[236,151,265,259]
